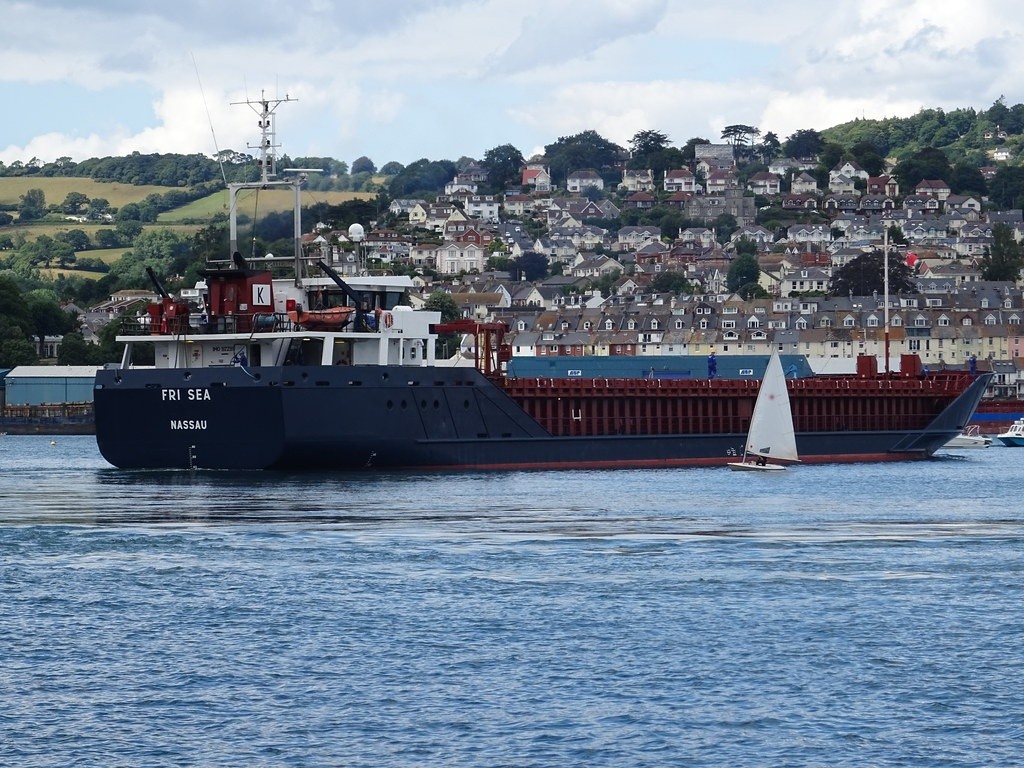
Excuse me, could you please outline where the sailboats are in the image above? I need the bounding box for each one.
[726,346,802,473]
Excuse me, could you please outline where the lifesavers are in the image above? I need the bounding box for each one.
[384,312,393,328]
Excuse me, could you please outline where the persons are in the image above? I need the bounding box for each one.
[708,349,718,379]
[921,363,932,381]
[969,352,978,375]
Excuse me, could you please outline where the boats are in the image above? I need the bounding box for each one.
[997,417,1024,447]
[93,85,997,473]
[943,423,993,448]
